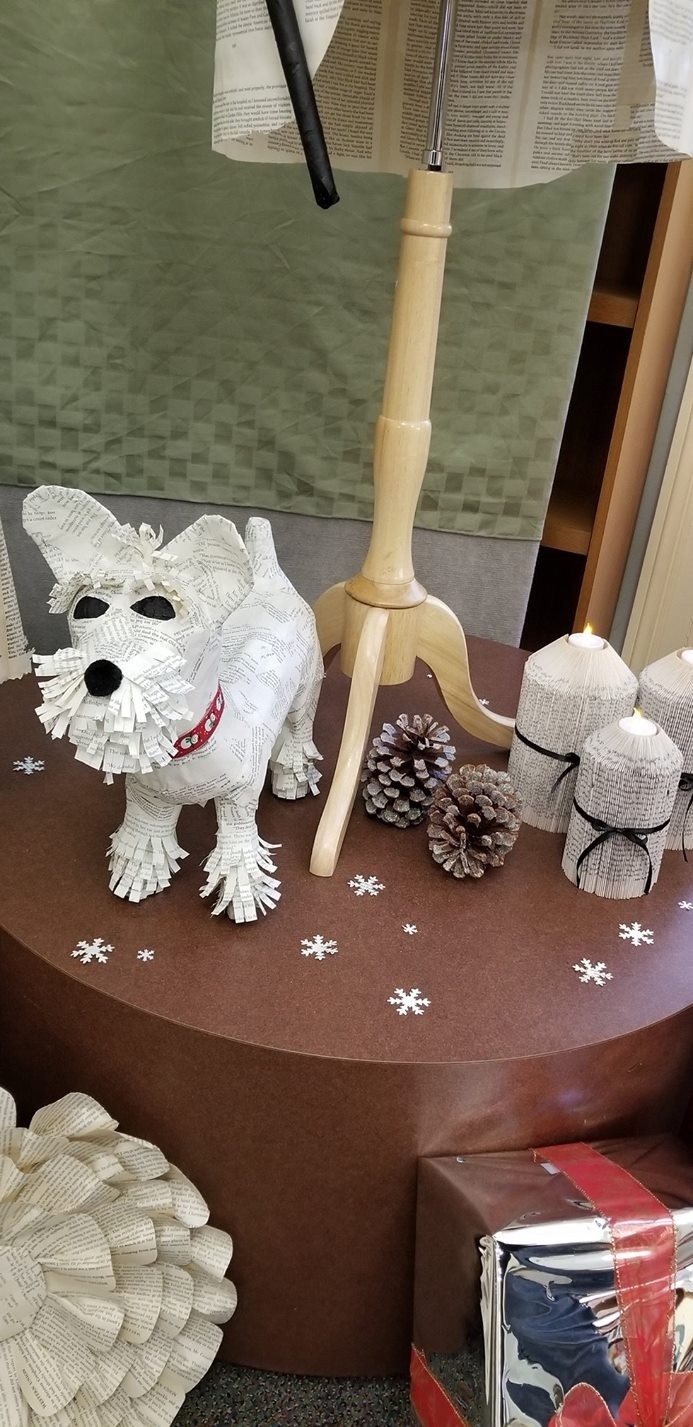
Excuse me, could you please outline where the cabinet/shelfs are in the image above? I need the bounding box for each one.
[541,159,693,641]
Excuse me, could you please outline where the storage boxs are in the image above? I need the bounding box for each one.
[406,1127,693,1427]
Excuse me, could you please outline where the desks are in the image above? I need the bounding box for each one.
[0,637,693,1381]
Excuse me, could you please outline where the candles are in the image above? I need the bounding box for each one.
[568,623,604,649]
[680,649,693,665]
[619,706,657,735]
